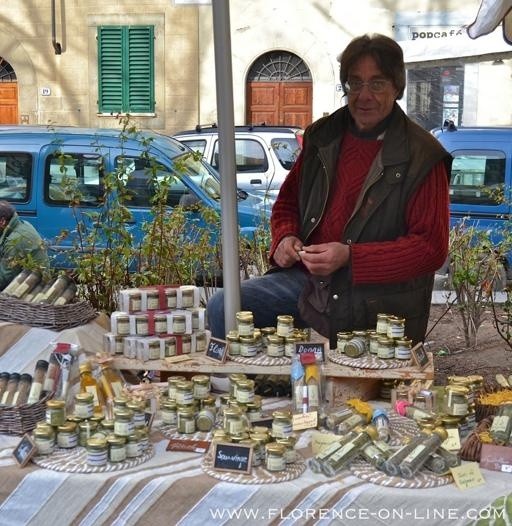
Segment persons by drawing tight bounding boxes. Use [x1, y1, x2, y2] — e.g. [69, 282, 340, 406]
[207, 33, 454, 348]
[0, 200, 50, 291]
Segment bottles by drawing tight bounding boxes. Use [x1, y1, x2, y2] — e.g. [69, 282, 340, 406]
[0, 269, 512, 478]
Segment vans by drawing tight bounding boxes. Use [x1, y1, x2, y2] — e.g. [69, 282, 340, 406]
[0, 126, 273, 273]
[420, 120, 511, 291]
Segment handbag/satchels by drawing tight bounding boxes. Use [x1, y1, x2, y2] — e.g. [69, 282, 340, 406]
[297, 273, 331, 339]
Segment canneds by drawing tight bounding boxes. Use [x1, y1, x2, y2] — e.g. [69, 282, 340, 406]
[0, 267, 512, 478]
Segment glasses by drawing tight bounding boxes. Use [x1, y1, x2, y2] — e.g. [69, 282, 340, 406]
[342, 77, 393, 96]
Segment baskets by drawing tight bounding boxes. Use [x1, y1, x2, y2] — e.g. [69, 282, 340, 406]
[0, 389, 56, 437]
[0, 294, 100, 332]
[474, 386, 512, 421]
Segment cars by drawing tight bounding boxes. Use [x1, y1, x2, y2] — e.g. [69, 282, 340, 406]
[170, 122, 305, 205]
